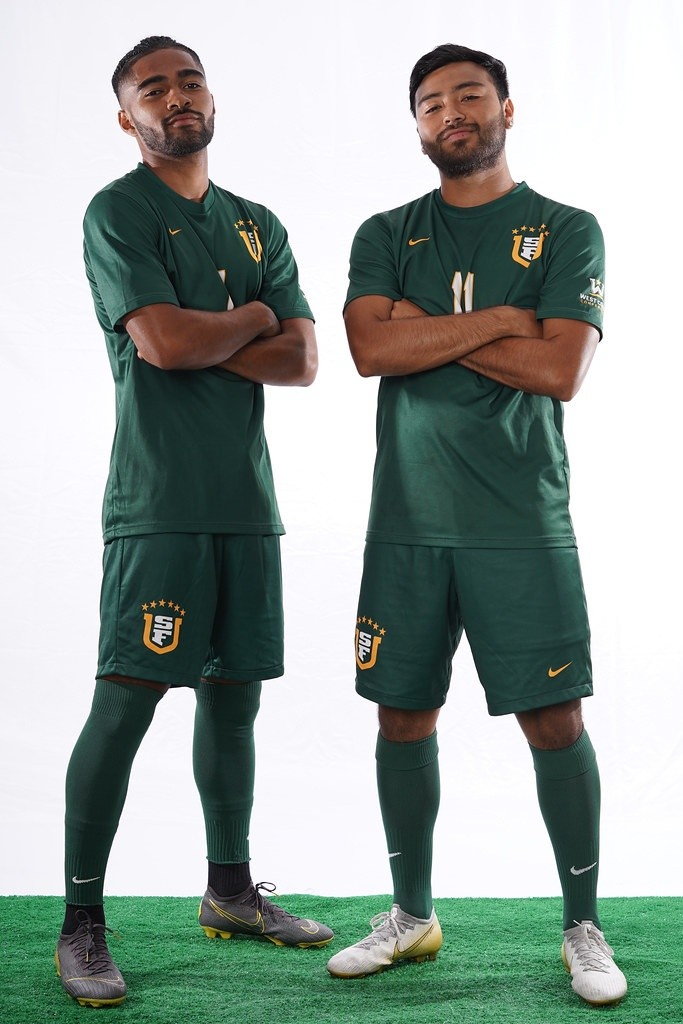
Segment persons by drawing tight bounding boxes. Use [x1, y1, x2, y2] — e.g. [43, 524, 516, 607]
[57, 36, 334, 1008]
[326, 45, 627, 1006]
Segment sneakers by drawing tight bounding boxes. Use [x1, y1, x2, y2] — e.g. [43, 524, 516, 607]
[327, 904, 442, 978]
[198, 884, 336, 948]
[53, 926, 127, 1008]
[560, 919, 627, 1004]
